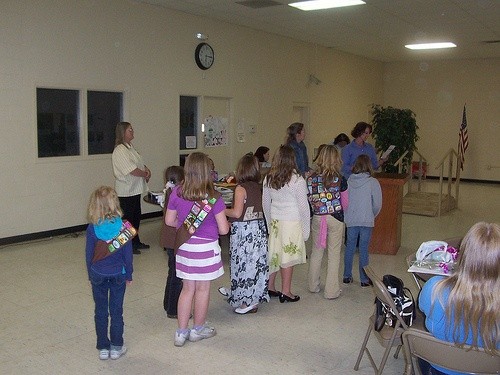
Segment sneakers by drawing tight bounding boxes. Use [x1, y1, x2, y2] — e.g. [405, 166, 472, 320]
[190, 327, 216, 340]
[174, 330, 190, 346]
[110, 346, 126, 359]
[99, 349, 110, 359]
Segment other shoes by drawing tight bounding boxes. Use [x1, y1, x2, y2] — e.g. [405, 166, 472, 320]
[167, 312, 193, 319]
[361, 280, 372, 287]
[133, 249, 140, 254]
[342, 278, 353, 284]
[138, 243, 149, 249]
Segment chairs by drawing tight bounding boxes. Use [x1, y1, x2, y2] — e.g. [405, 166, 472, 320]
[402, 328, 500, 375]
[353, 266, 430, 375]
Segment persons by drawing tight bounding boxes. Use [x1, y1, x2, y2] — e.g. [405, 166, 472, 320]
[159, 166, 193, 319]
[344, 154, 382, 288]
[254, 146, 272, 184]
[286, 122, 313, 179]
[307, 144, 348, 299]
[333, 133, 350, 147]
[86, 186, 133, 360]
[263, 144, 310, 303]
[341, 122, 389, 180]
[218, 154, 270, 314]
[417, 222, 500, 375]
[112, 122, 151, 255]
[165, 152, 232, 347]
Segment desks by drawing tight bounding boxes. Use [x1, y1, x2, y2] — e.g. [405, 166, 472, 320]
[368, 172, 410, 256]
[406, 235, 463, 283]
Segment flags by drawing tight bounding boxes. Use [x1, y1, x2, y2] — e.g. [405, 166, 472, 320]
[458, 105, 469, 171]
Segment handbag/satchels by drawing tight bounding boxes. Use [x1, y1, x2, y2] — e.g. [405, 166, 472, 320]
[374, 275, 415, 331]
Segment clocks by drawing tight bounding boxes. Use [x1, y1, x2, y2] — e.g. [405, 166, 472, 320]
[195, 43, 214, 70]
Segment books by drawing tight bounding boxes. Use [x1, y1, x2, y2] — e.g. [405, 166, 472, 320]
[381, 145, 395, 159]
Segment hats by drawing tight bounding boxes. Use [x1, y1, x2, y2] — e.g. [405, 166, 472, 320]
[408, 240, 457, 276]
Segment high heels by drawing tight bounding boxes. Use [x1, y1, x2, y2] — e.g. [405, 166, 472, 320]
[234, 303, 257, 314]
[218, 287, 228, 296]
[279, 292, 300, 303]
[268, 290, 280, 298]
